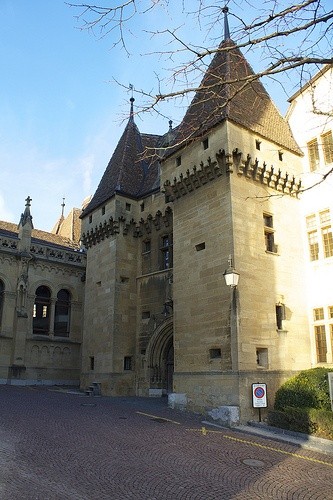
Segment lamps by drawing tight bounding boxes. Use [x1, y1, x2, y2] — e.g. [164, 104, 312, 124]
[223, 252, 240, 288]
[169, 120, 174, 127]
